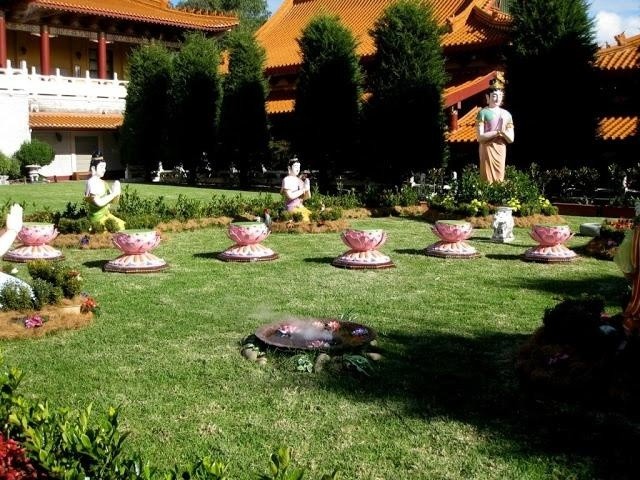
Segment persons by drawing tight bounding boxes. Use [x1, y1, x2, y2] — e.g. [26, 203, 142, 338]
[280, 153, 314, 215]
[0, 202, 40, 301]
[476, 80, 517, 180]
[85, 149, 125, 231]
